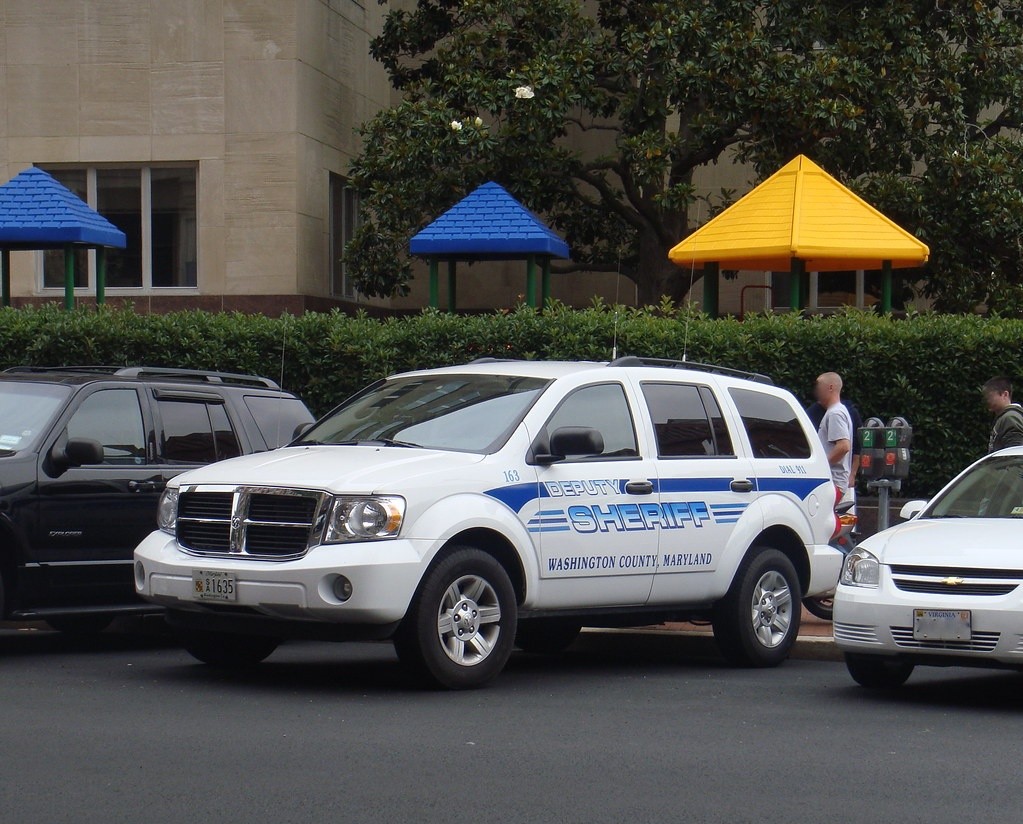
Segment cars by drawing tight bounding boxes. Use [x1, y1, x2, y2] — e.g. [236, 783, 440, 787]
[832, 446, 1023, 689]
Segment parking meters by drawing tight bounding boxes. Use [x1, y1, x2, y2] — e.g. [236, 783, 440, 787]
[857, 416, 912, 532]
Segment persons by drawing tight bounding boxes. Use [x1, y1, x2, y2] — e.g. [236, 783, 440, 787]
[978, 375, 1023, 517]
[806, 401, 864, 532]
[815, 372, 852, 499]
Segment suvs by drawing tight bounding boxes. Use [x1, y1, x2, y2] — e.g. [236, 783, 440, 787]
[132, 353, 845, 691]
[0, 363, 317, 649]
